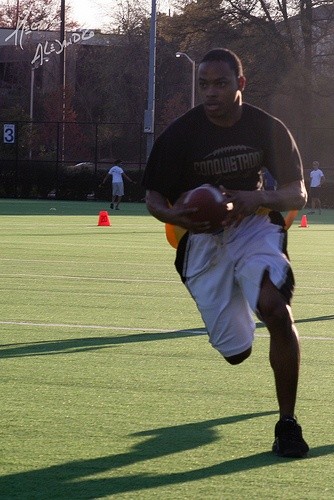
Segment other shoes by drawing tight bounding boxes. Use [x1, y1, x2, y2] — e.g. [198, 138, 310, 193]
[111, 204, 114, 209]
[116, 205, 119, 210]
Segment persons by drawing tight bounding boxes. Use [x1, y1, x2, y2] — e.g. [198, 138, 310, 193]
[262, 167, 277, 191]
[101, 160, 132, 210]
[308, 162, 326, 215]
[145, 49, 311, 456]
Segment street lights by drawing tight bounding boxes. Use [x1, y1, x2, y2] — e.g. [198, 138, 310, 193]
[175, 51, 195, 111]
[29, 56, 50, 159]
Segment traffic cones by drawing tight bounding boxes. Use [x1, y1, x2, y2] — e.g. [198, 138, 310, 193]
[98, 210, 112, 227]
[298, 214, 309, 228]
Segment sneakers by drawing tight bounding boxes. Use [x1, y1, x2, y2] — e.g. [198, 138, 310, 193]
[273, 421, 310, 458]
